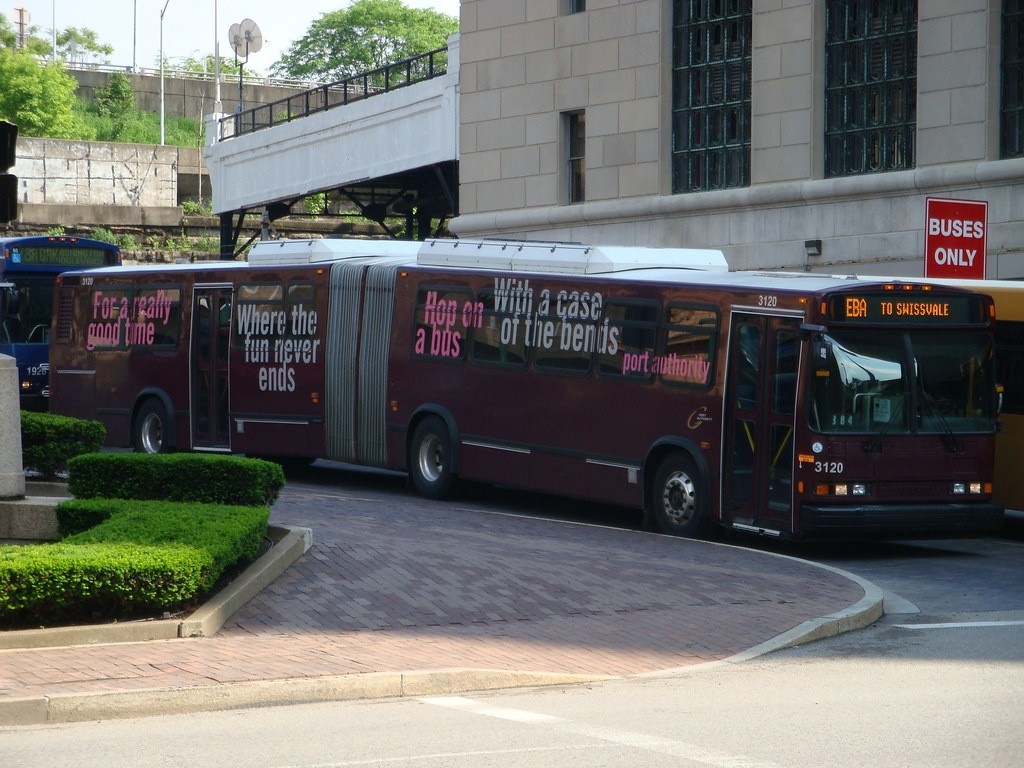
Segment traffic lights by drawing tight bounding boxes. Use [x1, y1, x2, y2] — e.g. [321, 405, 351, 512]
[0, 120, 20, 224]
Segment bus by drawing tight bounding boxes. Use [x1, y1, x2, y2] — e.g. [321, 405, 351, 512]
[833, 271, 1024, 533]
[0, 236, 123, 410]
[49, 239, 1010, 543]
